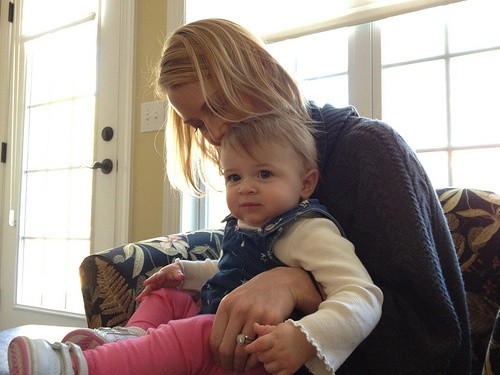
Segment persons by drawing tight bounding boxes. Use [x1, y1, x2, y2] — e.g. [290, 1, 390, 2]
[147, 18, 474, 375]
[7, 111, 384, 375]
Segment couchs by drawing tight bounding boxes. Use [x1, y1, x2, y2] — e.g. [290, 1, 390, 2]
[80, 188, 499, 375]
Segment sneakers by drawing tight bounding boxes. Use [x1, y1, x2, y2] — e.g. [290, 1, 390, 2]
[7, 326, 149, 375]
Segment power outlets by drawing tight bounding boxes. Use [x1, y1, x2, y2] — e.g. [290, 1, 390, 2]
[140, 100, 165, 133]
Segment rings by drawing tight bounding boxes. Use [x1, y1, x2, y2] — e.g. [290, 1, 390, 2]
[236, 334, 256, 345]
